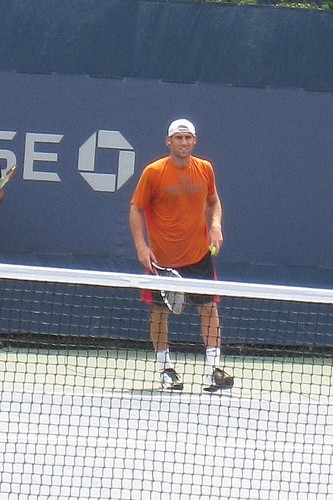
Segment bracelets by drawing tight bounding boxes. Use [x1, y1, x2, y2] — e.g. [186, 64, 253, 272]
[210, 223, 221, 231]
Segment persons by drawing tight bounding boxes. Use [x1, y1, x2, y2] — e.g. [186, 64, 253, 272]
[127, 118, 235, 391]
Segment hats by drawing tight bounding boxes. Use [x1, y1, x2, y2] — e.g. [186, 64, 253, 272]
[167, 119, 196, 136]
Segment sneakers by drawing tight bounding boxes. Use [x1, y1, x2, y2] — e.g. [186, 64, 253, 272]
[203, 368, 233, 392]
[159, 368, 182, 390]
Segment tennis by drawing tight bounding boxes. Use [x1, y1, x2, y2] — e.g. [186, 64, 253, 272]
[209, 246, 217, 257]
